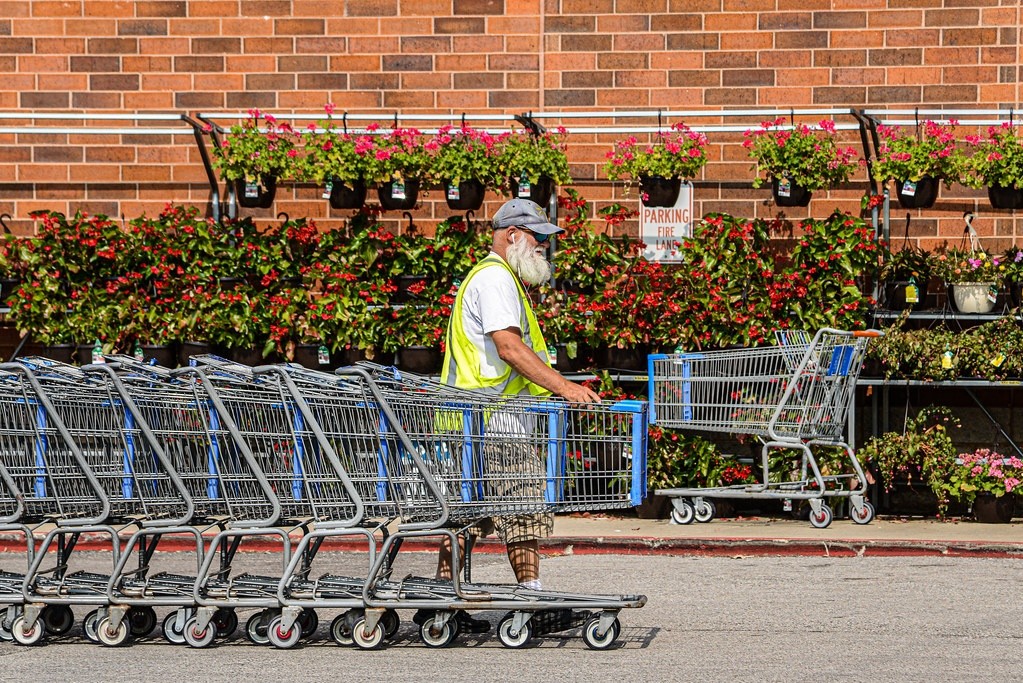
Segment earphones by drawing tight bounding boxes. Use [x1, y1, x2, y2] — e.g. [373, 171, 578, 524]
[511, 233, 515, 243]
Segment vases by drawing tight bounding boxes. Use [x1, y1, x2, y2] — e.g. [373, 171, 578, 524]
[0, 165, 1023, 525]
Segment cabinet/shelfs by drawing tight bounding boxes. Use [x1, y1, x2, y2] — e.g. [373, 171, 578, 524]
[365, 312, 1021, 518]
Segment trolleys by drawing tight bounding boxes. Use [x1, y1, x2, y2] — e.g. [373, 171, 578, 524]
[0, 349, 649, 649]
[647, 324, 889, 528]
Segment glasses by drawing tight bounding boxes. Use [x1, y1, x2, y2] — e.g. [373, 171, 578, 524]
[533, 231, 550, 242]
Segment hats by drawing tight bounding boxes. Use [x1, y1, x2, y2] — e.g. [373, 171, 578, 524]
[492, 197, 565, 235]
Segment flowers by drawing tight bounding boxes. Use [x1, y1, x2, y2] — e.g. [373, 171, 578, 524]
[0, 99, 1023, 524]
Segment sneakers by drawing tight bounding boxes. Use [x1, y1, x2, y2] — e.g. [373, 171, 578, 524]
[413, 605, 490, 635]
[531, 608, 591, 636]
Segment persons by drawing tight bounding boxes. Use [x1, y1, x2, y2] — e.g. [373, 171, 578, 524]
[412, 197, 603, 639]
